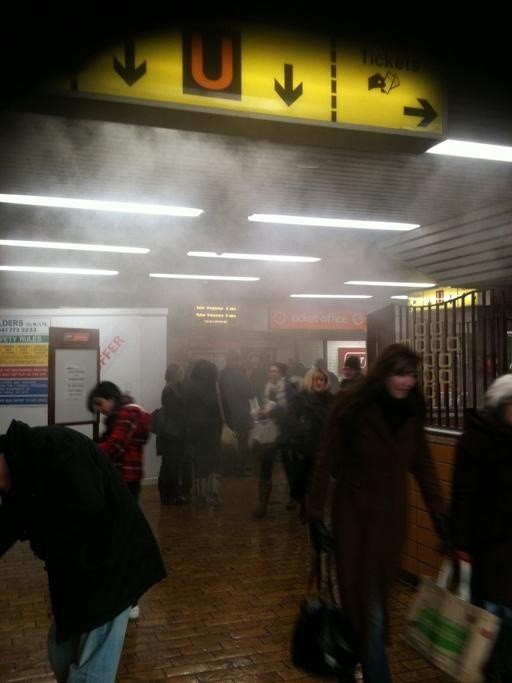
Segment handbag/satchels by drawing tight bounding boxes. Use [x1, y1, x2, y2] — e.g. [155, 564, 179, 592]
[150, 408, 184, 444]
[220, 424, 240, 458]
[247, 419, 282, 452]
[292, 596, 359, 683]
[397, 573, 504, 683]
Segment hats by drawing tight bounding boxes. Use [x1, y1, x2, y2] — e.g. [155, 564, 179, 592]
[482, 374, 512, 409]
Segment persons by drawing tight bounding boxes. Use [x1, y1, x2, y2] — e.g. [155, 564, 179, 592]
[303, 339, 454, 682]
[449, 370, 512, 683]
[155, 345, 364, 528]
[0, 415, 166, 683]
[84, 379, 150, 624]
